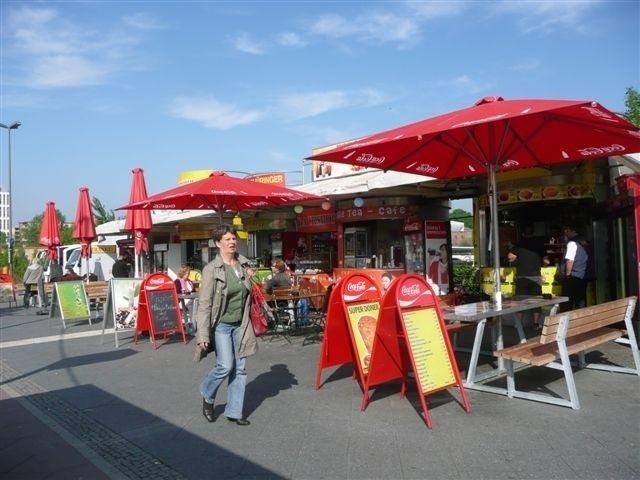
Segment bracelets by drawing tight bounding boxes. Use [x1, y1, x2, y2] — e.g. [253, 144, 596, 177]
[565, 274, 572, 278]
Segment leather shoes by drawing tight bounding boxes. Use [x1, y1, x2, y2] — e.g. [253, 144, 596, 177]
[203, 397, 215, 422]
[227, 416, 250, 425]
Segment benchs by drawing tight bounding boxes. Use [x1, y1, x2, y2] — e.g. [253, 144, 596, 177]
[25, 285, 640, 410]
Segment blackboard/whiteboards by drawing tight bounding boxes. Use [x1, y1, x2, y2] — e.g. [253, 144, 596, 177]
[144, 285, 183, 334]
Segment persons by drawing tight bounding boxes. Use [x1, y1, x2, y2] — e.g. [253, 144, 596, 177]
[62, 267, 78, 277]
[111, 251, 132, 278]
[559, 222, 594, 314]
[171, 266, 199, 315]
[46, 258, 63, 283]
[21, 257, 45, 310]
[242, 247, 311, 332]
[378, 273, 394, 299]
[427, 242, 450, 286]
[507, 220, 551, 330]
[191, 222, 259, 428]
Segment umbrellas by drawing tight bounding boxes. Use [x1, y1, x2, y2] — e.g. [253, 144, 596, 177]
[70, 186, 98, 309]
[304, 92, 640, 369]
[123, 166, 153, 277]
[38, 201, 64, 261]
[112, 169, 332, 227]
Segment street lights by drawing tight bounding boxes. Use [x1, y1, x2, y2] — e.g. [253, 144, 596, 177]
[0, 121, 22, 280]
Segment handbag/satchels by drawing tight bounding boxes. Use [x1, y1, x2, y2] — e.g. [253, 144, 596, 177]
[250, 282, 277, 337]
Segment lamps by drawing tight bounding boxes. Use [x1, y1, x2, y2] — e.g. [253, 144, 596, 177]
[321, 198, 331, 211]
[294, 205, 304, 214]
[353, 196, 365, 209]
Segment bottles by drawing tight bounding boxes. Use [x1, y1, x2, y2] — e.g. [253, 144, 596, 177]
[427, 248, 440, 257]
